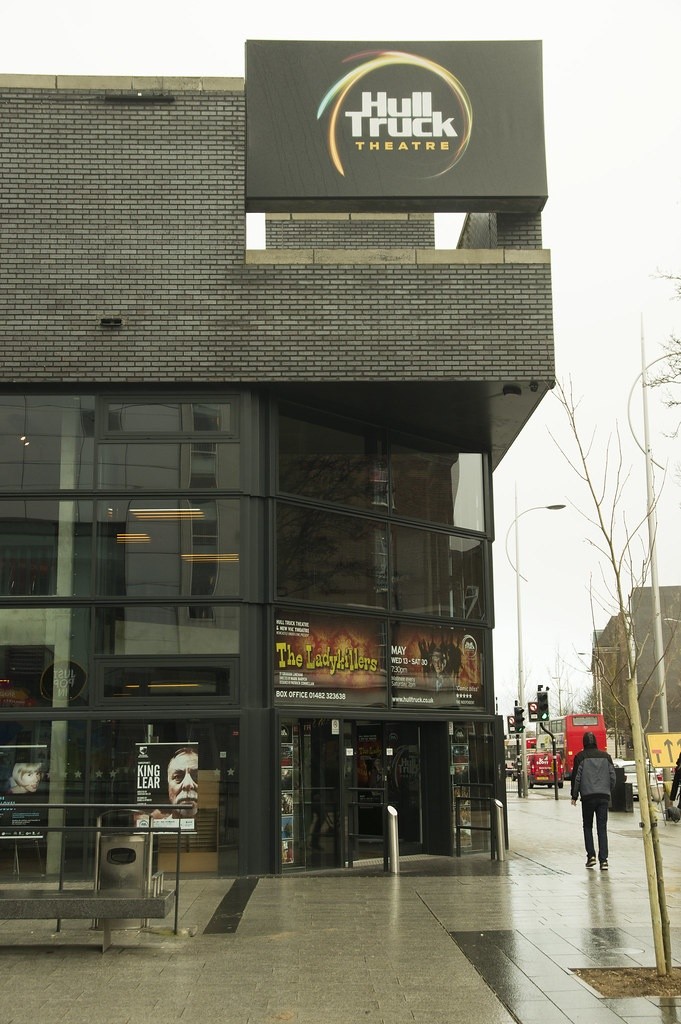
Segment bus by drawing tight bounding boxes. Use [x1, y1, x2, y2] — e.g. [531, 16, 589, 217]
[536, 714, 607, 781]
[505, 734, 537, 775]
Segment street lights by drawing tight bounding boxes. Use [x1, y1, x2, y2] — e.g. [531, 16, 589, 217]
[504, 480, 566, 800]
[578, 651, 604, 715]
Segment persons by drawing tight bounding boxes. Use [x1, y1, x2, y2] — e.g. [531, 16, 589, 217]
[669, 752, 681, 810]
[7, 763, 46, 799]
[432, 650, 453, 691]
[570, 732, 616, 870]
[168, 747, 199, 822]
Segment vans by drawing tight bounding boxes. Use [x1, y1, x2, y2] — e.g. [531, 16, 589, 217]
[526, 751, 563, 788]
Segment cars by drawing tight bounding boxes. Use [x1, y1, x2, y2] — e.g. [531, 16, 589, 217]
[614, 758, 675, 800]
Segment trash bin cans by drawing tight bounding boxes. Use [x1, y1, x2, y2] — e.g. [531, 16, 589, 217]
[613, 767, 634, 813]
[100, 833, 149, 931]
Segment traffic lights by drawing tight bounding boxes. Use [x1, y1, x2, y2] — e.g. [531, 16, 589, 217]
[537, 692, 550, 721]
[514, 706, 525, 733]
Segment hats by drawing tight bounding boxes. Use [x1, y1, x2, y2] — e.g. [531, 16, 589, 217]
[583, 732, 596, 745]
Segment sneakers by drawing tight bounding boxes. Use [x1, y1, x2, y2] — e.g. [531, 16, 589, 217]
[600, 859, 609, 869]
[586, 853, 596, 867]
